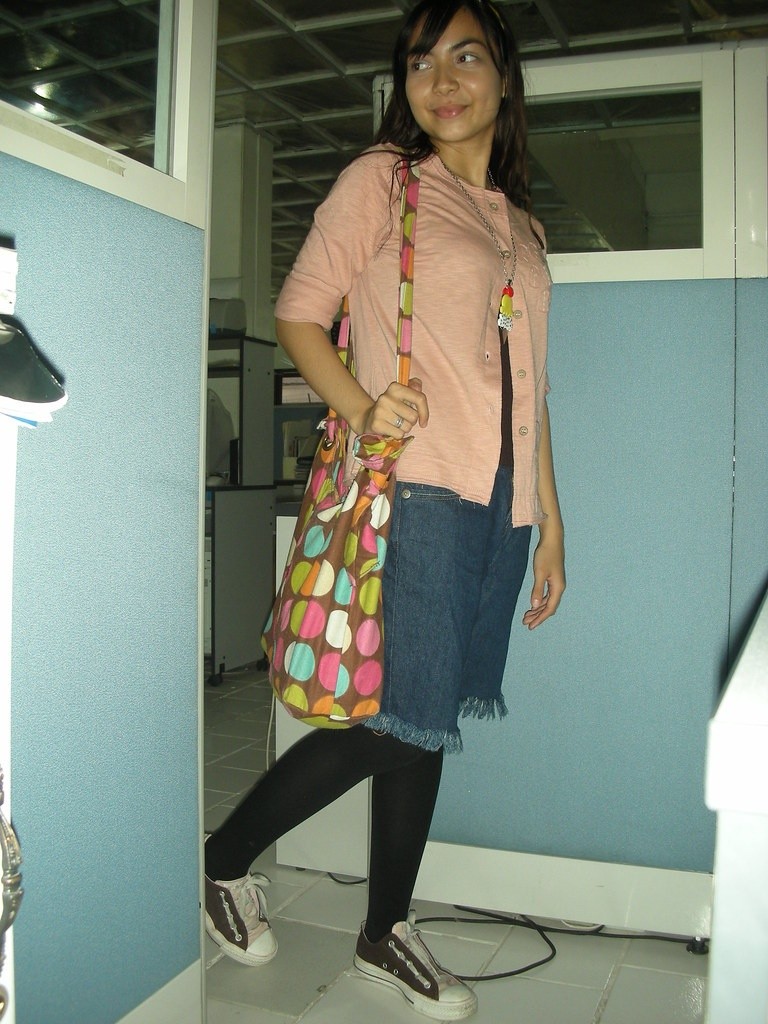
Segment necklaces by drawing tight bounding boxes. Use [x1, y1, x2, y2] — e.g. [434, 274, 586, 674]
[440, 159, 517, 331]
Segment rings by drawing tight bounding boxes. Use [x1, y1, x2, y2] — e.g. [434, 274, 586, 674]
[396, 418, 404, 429]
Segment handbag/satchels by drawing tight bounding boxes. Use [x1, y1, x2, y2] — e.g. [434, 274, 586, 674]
[260, 408, 414, 730]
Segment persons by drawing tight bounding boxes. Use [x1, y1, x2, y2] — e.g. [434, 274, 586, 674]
[205, 1, 565, 1022]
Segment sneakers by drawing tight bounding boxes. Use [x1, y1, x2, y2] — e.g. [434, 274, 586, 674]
[352, 909, 478, 1021]
[202, 833, 279, 967]
